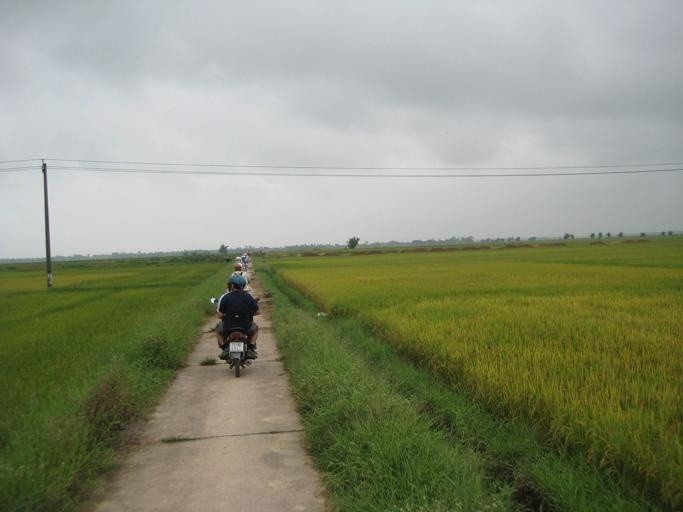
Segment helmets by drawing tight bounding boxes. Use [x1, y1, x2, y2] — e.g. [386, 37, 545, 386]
[228, 253, 247, 285]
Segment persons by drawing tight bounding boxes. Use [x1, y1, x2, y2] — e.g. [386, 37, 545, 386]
[215, 252, 263, 359]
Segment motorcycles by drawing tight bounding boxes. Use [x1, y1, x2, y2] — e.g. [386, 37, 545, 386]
[211, 297, 260, 377]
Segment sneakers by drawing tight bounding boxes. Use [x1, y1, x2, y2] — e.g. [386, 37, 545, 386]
[219, 349, 228, 358]
[246, 349, 257, 357]
